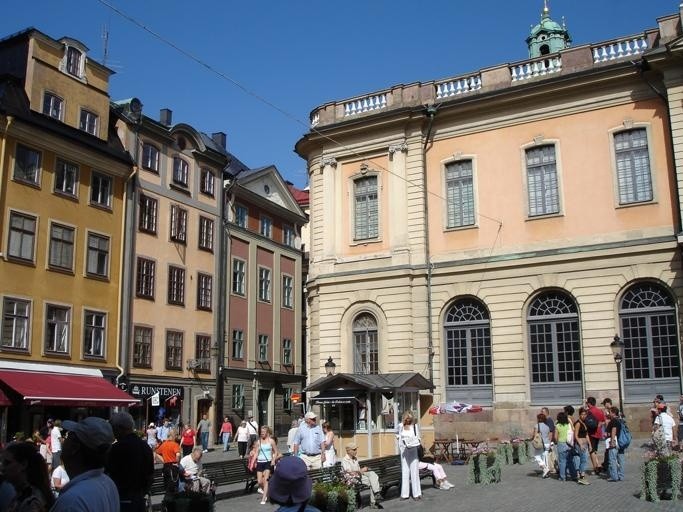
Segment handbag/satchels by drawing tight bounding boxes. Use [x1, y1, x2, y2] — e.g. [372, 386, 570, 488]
[248, 455, 257, 470]
[418, 445, 423, 460]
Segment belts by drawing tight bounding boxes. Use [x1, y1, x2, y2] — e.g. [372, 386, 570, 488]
[303, 453, 321, 456]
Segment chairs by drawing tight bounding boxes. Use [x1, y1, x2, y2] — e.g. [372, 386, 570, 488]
[431, 437, 483, 464]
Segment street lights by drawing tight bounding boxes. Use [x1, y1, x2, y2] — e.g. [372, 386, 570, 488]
[324, 356, 336, 429]
[609, 333, 625, 418]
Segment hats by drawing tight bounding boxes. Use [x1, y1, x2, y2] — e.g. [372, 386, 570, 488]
[346, 443, 359, 450]
[268, 456, 312, 503]
[62, 417, 114, 453]
[305, 412, 318, 419]
[148, 422, 156, 428]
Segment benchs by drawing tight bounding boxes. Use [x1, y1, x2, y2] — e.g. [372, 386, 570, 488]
[143, 452, 294, 512]
[307, 453, 437, 508]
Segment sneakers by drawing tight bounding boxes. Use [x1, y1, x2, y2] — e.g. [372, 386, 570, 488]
[444, 480, 455, 488]
[440, 482, 450, 490]
[375, 492, 384, 500]
[257, 488, 267, 505]
[370, 503, 381, 509]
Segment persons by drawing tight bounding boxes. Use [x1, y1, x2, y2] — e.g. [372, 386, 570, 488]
[533, 397, 625, 485]
[341, 443, 384, 509]
[220, 417, 232, 453]
[287, 412, 336, 487]
[394, 410, 455, 501]
[233, 417, 282, 505]
[0, 409, 216, 512]
[651, 395, 682, 455]
